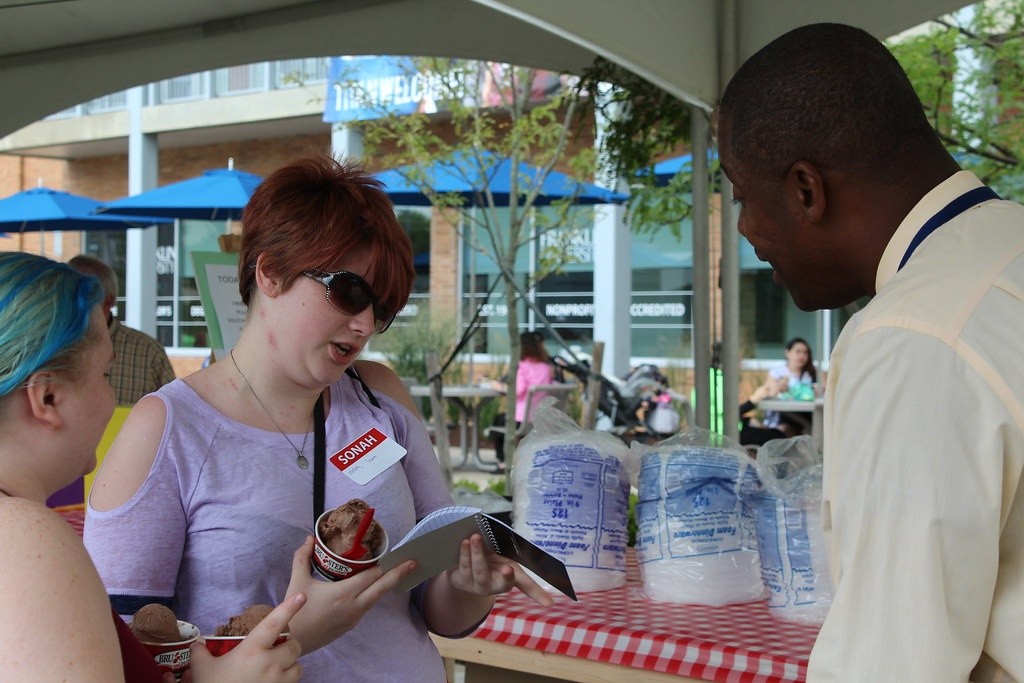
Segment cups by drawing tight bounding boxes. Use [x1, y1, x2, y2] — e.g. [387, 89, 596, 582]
[310, 508, 389, 582]
[203, 632, 290, 657]
[128, 621, 200, 683]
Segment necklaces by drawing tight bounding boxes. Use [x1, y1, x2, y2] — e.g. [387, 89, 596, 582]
[230, 348, 312, 470]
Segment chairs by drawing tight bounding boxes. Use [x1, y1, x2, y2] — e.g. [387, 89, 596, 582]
[665, 388, 761, 458]
[484, 384, 579, 439]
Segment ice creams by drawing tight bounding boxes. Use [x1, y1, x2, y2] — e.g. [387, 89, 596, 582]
[312, 497, 389, 583]
[120, 604, 290, 683]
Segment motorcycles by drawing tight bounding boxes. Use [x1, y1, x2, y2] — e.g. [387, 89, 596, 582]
[558, 354, 669, 448]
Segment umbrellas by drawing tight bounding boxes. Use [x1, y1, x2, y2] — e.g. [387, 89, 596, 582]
[0, 157, 263, 255]
[362, 137, 636, 387]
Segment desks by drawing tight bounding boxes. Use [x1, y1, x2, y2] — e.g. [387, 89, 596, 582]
[408, 387, 505, 471]
[429, 546, 824, 683]
[760, 400, 814, 435]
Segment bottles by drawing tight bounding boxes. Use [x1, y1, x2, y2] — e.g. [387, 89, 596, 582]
[800, 371, 815, 401]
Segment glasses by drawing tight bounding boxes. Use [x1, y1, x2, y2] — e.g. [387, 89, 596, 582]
[303, 269, 403, 335]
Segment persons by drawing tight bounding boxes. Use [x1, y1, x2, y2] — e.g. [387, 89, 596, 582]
[719, 23, 1024, 683]
[82, 146, 555, 683]
[68, 254, 176, 407]
[490, 328, 560, 475]
[0, 255, 313, 683]
[690, 336, 830, 448]
